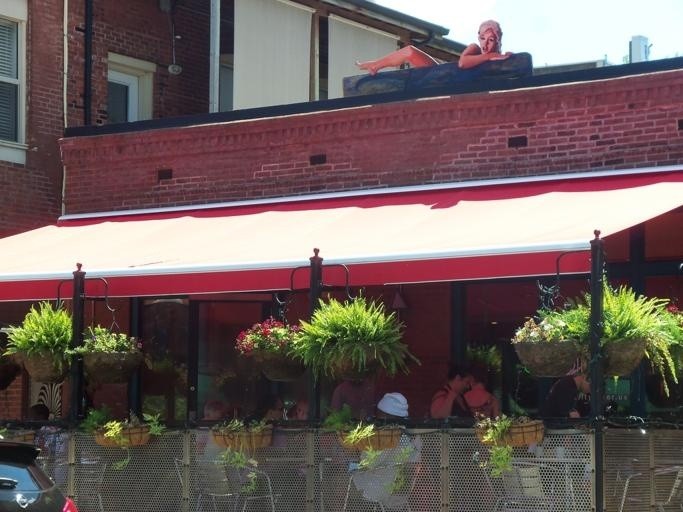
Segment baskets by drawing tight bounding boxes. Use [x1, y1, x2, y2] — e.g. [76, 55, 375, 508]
[213, 430, 273, 449]
[475, 421, 545, 445]
[254, 349, 307, 383]
[94, 424, 154, 447]
[22, 347, 69, 384]
[329, 355, 384, 381]
[336, 432, 401, 451]
[584, 341, 647, 380]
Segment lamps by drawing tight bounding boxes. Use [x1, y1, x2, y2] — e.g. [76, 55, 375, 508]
[158, 1, 182, 75]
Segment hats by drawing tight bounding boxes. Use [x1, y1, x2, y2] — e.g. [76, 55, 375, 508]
[377, 392, 409, 417]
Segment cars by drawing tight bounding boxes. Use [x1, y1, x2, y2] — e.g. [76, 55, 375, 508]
[0, 436, 79, 512]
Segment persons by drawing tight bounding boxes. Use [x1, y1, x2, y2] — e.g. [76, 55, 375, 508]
[429, 370, 500, 418]
[201, 399, 311, 489]
[354, 20, 513, 77]
[542, 375, 596, 419]
[29, 404, 49, 420]
[348, 392, 421, 503]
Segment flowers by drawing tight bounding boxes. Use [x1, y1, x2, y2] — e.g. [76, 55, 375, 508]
[510, 315, 578, 345]
[64, 324, 143, 357]
[232, 316, 309, 358]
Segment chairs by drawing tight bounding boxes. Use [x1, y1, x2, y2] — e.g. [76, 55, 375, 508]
[0, 421, 683, 510]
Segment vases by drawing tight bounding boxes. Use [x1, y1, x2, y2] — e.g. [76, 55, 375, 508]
[245, 351, 285, 382]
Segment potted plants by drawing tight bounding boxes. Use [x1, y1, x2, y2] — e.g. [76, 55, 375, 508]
[1, 298, 72, 382]
[472, 412, 545, 480]
[78, 404, 168, 470]
[535, 276, 683, 398]
[319, 403, 414, 494]
[285, 290, 421, 384]
[210, 418, 273, 496]
